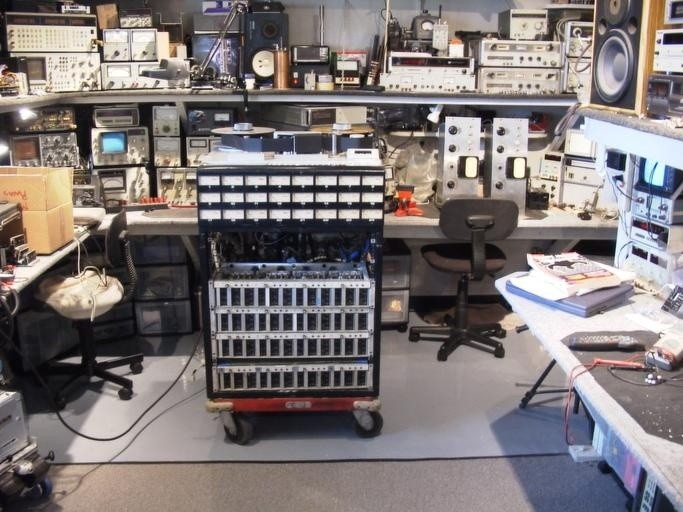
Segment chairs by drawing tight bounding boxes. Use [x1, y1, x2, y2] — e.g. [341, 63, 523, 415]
[34, 212, 144, 412]
[410, 199, 519, 362]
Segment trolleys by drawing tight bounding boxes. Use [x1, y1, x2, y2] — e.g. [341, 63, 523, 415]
[196, 146, 382, 446]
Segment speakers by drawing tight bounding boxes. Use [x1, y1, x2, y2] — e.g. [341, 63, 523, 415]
[244, 11, 289, 85]
[589, 0, 666, 118]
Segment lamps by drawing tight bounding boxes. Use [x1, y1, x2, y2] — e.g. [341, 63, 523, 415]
[137, 2, 247, 110]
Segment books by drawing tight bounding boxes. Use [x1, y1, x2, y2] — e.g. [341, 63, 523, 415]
[528, 251, 619, 296]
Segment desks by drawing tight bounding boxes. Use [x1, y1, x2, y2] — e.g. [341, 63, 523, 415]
[2, 89, 616, 357]
[496, 265, 681, 512]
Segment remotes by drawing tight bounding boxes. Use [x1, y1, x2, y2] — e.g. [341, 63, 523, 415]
[568, 335, 645, 350]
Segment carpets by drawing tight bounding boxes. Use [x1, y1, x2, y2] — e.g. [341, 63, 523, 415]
[24, 327, 593, 464]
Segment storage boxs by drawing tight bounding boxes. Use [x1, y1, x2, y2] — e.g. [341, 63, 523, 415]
[135, 264, 190, 301]
[0, 165, 75, 255]
[135, 303, 192, 336]
[380, 289, 409, 331]
[134, 232, 188, 265]
[380, 237, 409, 289]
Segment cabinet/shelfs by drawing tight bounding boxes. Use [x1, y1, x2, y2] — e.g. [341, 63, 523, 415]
[577, 1, 682, 302]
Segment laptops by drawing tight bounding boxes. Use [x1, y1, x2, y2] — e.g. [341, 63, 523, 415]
[506, 271, 635, 318]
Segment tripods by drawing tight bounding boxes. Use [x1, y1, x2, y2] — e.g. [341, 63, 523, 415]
[518, 358, 595, 442]
